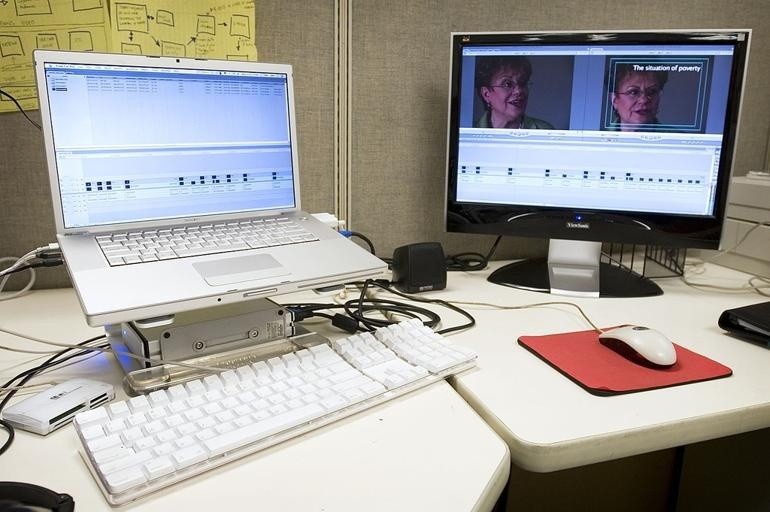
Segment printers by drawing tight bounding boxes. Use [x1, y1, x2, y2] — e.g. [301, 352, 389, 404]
[700, 170, 770, 279]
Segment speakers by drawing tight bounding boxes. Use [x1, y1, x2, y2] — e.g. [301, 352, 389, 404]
[392, 242, 447, 294]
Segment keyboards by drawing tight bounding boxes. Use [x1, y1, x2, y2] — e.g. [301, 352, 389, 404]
[70, 318, 479, 507]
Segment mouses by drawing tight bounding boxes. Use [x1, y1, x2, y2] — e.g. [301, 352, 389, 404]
[598, 325, 678, 370]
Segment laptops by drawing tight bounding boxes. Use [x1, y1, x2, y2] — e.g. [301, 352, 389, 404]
[33, 49, 389, 327]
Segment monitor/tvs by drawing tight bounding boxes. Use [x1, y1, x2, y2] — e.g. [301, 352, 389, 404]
[445, 29, 754, 299]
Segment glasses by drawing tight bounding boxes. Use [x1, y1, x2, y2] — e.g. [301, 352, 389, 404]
[488, 79, 535, 91]
[613, 87, 661, 98]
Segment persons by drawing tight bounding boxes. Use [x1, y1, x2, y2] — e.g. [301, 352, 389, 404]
[600, 61, 670, 132]
[474, 55, 556, 131]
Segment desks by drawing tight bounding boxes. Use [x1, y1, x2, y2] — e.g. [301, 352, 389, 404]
[0, 287, 512, 512]
[357, 250, 770, 512]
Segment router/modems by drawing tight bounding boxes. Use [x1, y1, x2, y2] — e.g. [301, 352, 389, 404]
[2, 378, 115, 437]
